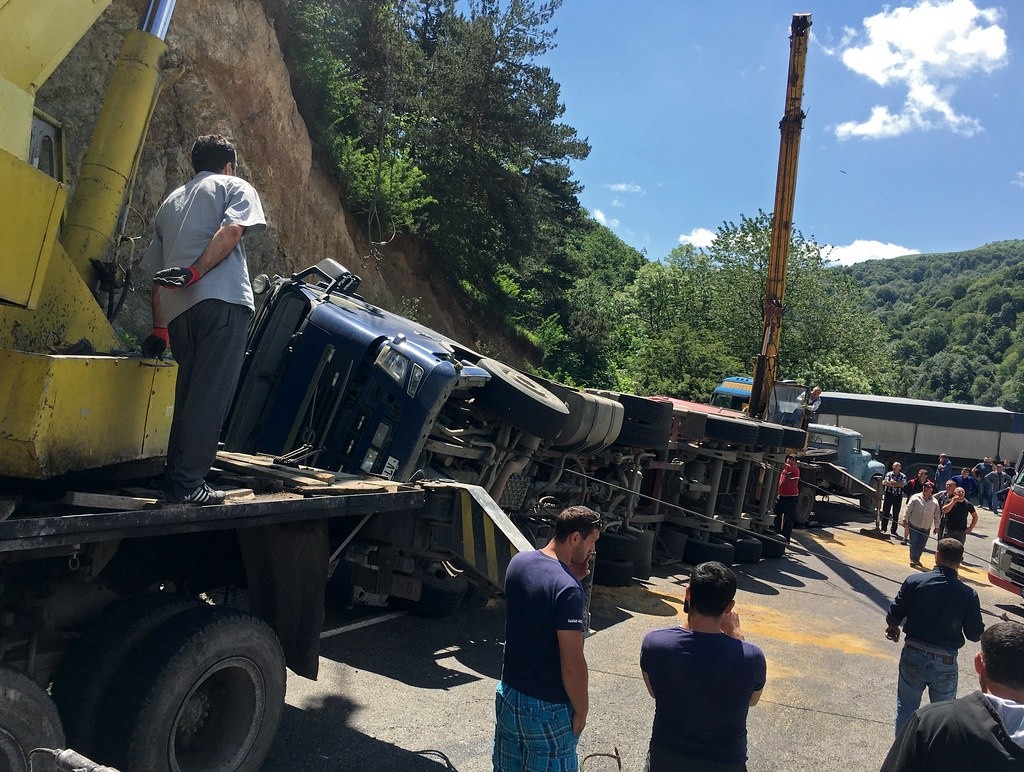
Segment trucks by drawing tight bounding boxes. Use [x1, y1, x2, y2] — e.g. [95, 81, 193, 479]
[794, 416, 887, 523]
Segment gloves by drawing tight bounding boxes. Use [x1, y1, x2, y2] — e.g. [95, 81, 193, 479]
[140, 327, 168, 361]
[800, 402, 808, 409]
[152, 266, 202, 290]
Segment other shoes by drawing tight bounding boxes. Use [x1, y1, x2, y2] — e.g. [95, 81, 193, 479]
[891, 531, 898, 536]
[880, 528, 886, 533]
[978, 504, 981, 508]
[900, 539, 908, 545]
[910, 561, 915, 566]
[989, 508, 993, 511]
[994, 511, 998, 514]
[914, 561, 922, 566]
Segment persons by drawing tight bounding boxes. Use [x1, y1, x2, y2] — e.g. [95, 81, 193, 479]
[971, 456, 1015, 515]
[903, 470, 978, 566]
[492, 506, 605, 772]
[886, 538, 984, 738]
[772, 454, 799, 542]
[934, 454, 953, 492]
[880, 462, 907, 535]
[141, 136, 266, 505]
[640, 561, 766, 772]
[796, 386, 822, 428]
[950, 468, 978, 502]
[879, 622, 1024, 772]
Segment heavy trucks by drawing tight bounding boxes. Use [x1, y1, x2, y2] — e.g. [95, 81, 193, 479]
[0, 0, 885, 772]
[705, 376, 1024, 484]
[988, 450, 1024, 598]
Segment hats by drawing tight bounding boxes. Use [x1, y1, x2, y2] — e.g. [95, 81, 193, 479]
[924, 481, 935, 489]
[790, 453, 798, 462]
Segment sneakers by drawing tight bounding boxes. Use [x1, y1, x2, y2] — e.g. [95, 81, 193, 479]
[167, 482, 227, 506]
[142, 472, 176, 493]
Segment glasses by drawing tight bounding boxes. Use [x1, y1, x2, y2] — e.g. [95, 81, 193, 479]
[589, 514, 603, 527]
